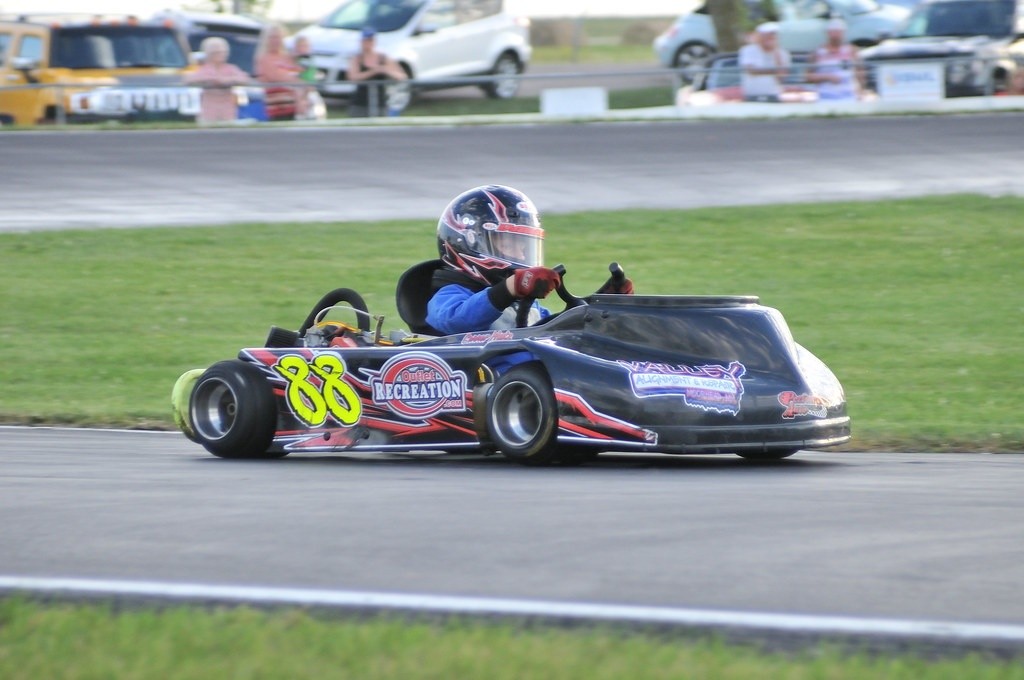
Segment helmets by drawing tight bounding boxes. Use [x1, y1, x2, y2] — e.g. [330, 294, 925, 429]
[435, 184, 544, 286]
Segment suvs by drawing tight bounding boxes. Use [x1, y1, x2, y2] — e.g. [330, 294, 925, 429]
[284, 0, 532, 114]
[0, 13, 264, 129]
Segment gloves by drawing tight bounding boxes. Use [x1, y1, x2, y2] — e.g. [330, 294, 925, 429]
[596, 277, 635, 294]
[515, 266, 561, 300]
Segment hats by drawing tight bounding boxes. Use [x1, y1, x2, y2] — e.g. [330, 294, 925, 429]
[756, 20, 778, 33]
[361, 26, 378, 38]
[822, 17, 845, 30]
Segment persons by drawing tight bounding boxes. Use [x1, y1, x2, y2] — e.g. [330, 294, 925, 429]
[424, 185, 635, 334]
[737, 22, 792, 101]
[184, 36, 256, 121]
[348, 26, 407, 118]
[261, 25, 326, 121]
[293, 37, 312, 70]
[806, 18, 866, 101]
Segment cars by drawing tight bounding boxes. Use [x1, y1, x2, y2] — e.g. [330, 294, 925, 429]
[653, 0, 1024, 106]
